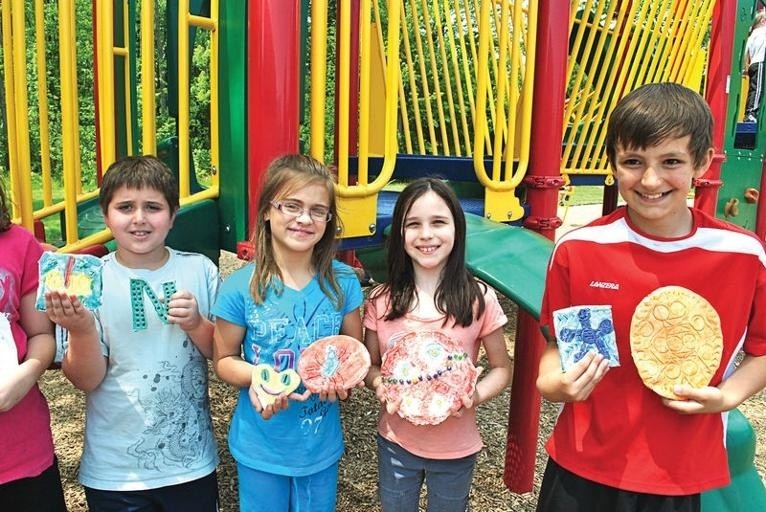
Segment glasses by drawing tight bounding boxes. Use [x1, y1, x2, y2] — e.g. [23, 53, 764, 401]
[268, 199, 333, 224]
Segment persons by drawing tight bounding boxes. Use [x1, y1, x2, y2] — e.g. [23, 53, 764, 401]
[208, 153, 366, 511]
[744, 12, 766, 122]
[44, 155, 224, 512]
[0, 174, 68, 510]
[533, 82, 766, 511]
[358, 176, 512, 511]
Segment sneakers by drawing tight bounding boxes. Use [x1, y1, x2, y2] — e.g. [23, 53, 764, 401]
[743, 114, 756, 122]
